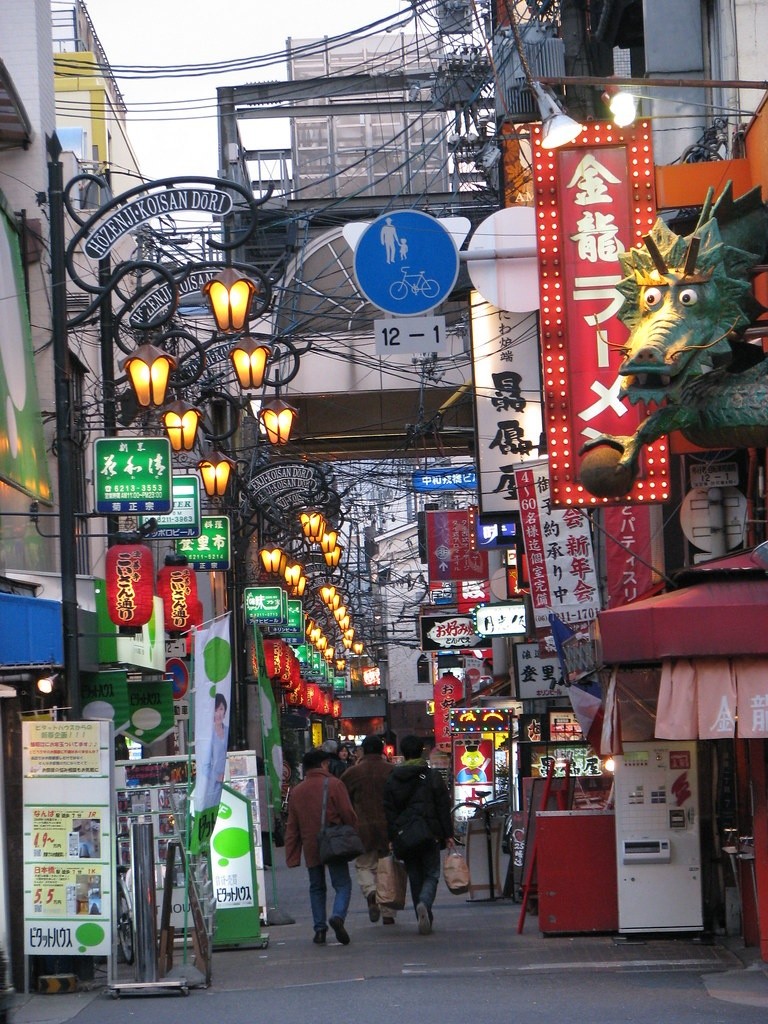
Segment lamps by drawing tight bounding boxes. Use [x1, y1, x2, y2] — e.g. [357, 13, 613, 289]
[601, 85, 640, 126]
[37, 674, 59, 693]
[533, 86, 583, 149]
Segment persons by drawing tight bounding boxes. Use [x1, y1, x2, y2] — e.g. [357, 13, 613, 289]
[204, 693, 229, 808]
[282, 733, 456, 946]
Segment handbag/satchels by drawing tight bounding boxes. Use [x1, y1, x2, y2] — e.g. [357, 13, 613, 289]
[317, 825, 365, 865]
[443, 839, 470, 895]
[376, 850, 408, 910]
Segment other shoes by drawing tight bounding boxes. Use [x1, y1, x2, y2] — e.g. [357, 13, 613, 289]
[367, 891, 380, 922]
[416, 901, 433, 935]
[313, 930, 326, 944]
[328, 917, 351, 946]
[383, 917, 395, 925]
